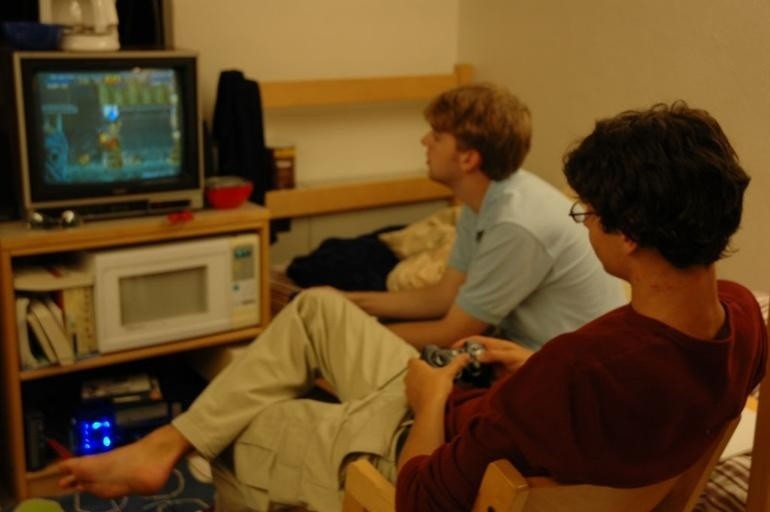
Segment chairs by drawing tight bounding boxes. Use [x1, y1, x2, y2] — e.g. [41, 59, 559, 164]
[337, 410, 741, 512]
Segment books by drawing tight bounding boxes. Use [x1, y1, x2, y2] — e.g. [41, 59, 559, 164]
[14, 266, 96, 369]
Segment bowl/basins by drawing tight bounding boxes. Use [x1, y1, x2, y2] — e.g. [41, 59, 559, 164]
[206, 177, 253, 210]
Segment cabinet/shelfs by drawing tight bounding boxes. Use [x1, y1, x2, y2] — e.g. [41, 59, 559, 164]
[0, 199, 271, 499]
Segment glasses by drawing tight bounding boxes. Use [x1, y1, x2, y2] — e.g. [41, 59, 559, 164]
[568, 201, 601, 226]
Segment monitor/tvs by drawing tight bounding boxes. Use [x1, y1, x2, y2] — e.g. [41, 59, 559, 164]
[13, 51, 204, 223]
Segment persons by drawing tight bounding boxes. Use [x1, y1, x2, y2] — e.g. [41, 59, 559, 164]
[57, 100, 764, 512]
[301, 82, 628, 369]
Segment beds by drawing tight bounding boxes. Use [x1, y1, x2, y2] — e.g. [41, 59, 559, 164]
[203, 62, 770, 510]
